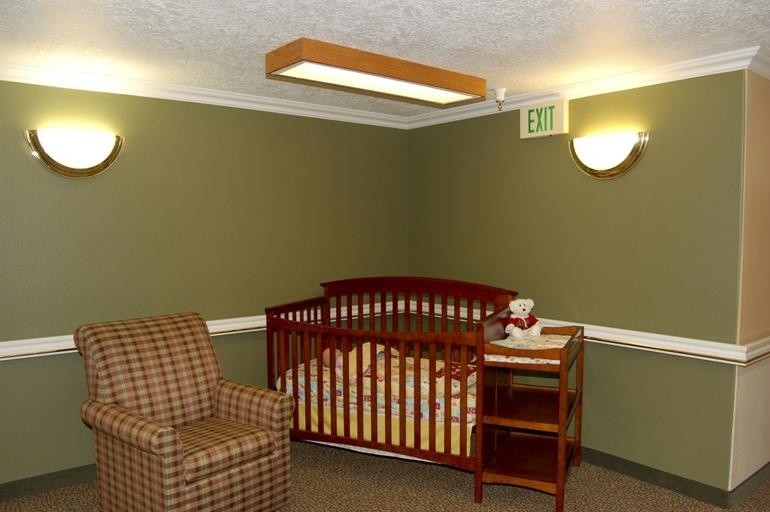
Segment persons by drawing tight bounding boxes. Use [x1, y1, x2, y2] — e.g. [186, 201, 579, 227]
[321, 349, 384, 385]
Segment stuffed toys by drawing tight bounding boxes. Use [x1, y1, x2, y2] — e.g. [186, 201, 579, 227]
[505, 298, 543, 338]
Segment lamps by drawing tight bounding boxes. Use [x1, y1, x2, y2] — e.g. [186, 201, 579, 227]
[27, 126, 126, 178]
[493, 87, 506, 112]
[569, 132, 650, 179]
[265, 37, 487, 110]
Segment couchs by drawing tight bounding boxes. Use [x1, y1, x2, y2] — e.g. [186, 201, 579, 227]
[74, 311, 297, 512]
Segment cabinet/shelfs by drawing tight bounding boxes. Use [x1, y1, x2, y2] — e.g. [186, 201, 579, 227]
[475, 325, 584, 512]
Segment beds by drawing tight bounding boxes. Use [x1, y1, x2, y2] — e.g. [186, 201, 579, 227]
[266, 275, 519, 473]
